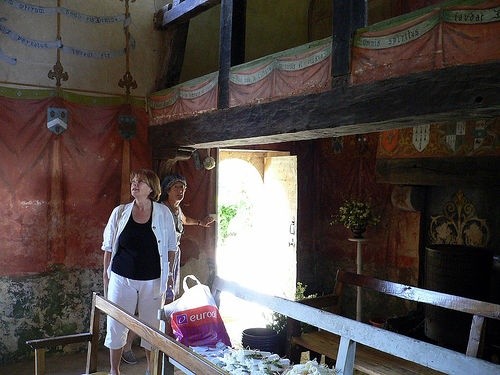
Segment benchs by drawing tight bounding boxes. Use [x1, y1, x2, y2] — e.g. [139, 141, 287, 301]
[24, 269, 500, 375]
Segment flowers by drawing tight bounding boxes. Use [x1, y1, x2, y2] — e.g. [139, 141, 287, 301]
[329, 198, 381, 228]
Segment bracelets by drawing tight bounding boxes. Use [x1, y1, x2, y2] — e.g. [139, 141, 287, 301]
[197, 220, 201, 226]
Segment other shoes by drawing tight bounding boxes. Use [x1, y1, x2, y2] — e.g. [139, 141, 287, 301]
[122, 350, 137, 364]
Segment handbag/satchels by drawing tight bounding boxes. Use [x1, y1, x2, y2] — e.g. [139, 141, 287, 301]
[162, 273, 232, 347]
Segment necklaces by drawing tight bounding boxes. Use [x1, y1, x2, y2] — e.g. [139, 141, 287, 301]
[168, 200, 178, 217]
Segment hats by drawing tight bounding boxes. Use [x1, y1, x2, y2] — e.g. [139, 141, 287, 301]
[162, 176, 187, 195]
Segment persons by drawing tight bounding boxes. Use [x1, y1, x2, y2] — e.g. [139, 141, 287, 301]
[101, 168, 179, 375]
[121, 173, 217, 365]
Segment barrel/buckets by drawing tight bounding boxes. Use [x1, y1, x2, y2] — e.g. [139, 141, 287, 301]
[424, 244, 484, 344]
[242, 328, 280, 356]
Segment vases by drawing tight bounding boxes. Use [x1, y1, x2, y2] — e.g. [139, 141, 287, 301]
[351, 226, 366, 239]
